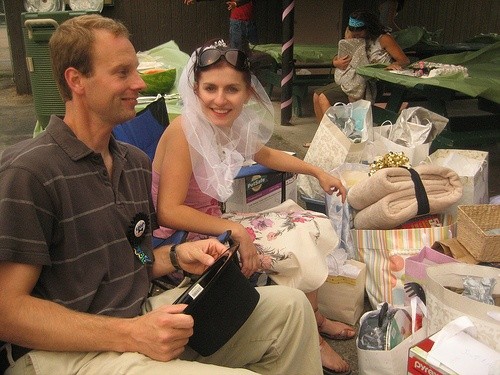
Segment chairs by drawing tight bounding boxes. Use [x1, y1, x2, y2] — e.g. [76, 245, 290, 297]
[112, 96, 295, 299]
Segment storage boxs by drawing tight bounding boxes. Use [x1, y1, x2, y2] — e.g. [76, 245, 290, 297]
[407, 326, 500, 375]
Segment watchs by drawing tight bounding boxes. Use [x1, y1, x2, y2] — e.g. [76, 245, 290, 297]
[169, 244, 182, 270]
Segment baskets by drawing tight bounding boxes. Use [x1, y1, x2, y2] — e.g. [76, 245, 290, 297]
[457, 204, 500, 263]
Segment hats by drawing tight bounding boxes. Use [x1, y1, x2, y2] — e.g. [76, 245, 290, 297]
[172, 242, 260, 357]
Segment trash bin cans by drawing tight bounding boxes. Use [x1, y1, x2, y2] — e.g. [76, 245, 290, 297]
[20, 9, 101, 129]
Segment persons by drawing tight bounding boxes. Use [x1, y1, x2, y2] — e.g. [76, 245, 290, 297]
[184, 0, 258, 50]
[0, 17, 324, 375]
[302, 9, 409, 148]
[151, 34, 358, 375]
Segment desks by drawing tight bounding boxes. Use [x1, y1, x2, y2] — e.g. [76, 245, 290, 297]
[132, 53, 192, 122]
[354, 51, 500, 132]
[250, 44, 490, 119]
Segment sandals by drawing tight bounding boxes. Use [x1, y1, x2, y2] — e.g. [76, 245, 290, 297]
[320, 338, 351, 375]
[318, 315, 355, 339]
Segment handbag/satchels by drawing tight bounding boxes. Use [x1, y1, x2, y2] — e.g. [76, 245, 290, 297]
[318, 254, 367, 326]
[294, 98, 449, 200]
[353, 226, 500, 372]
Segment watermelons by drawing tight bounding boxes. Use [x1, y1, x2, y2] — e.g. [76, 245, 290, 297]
[138, 68, 176, 95]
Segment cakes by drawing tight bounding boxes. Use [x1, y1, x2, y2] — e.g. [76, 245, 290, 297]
[409, 61, 467, 78]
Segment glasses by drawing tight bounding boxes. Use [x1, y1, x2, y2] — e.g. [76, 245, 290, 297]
[197, 49, 251, 72]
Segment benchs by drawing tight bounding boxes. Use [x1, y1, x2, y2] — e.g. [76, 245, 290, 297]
[260, 63, 500, 149]
